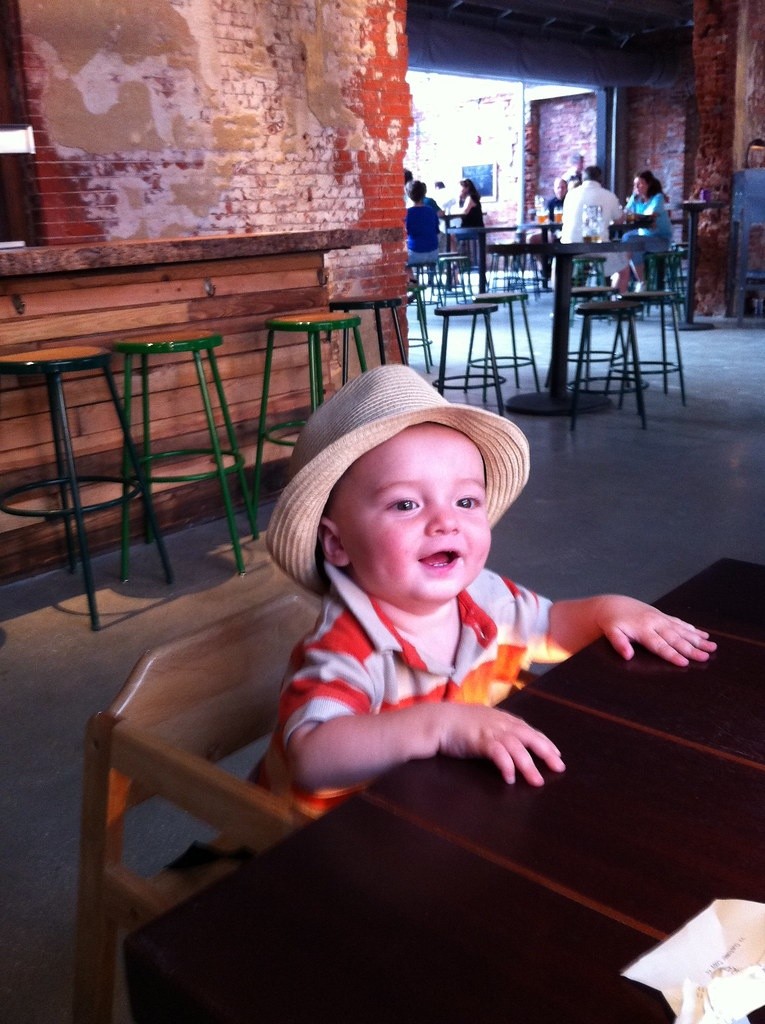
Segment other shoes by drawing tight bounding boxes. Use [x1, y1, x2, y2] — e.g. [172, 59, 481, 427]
[634, 280, 648, 293]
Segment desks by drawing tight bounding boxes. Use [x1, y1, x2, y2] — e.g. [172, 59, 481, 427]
[121, 556, 765, 1024]
[447, 225, 518, 294]
[435, 212, 467, 297]
[0, 226, 404, 294]
[470, 212, 488, 271]
[484, 240, 671, 418]
[517, 224, 563, 293]
[662, 201, 728, 331]
[607, 222, 652, 242]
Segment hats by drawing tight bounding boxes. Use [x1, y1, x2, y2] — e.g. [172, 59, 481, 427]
[265, 364, 530, 596]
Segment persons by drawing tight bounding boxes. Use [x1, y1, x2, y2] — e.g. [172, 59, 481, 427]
[560, 166, 628, 321]
[526, 179, 567, 280]
[405, 181, 441, 285]
[623, 170, 674, 292]
[562, 155, 585, 192]
[404, 167, 483, 286]
[217, 364, 717, 858]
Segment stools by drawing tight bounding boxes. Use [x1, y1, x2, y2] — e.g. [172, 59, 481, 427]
[0, 345, 175, 633]
[249, 314, 369, 521]
[110, 328, 260, 585]
[329, 295, 409, 387]
[407, 241, 689, 430]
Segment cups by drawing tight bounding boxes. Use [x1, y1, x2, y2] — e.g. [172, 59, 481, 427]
[554, 206, 564, 223]
[582, 204, 603, 244]
[536, 206, 549, 224]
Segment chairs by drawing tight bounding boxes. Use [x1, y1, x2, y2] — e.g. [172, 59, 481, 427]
[70, 593, 319, 1024]
